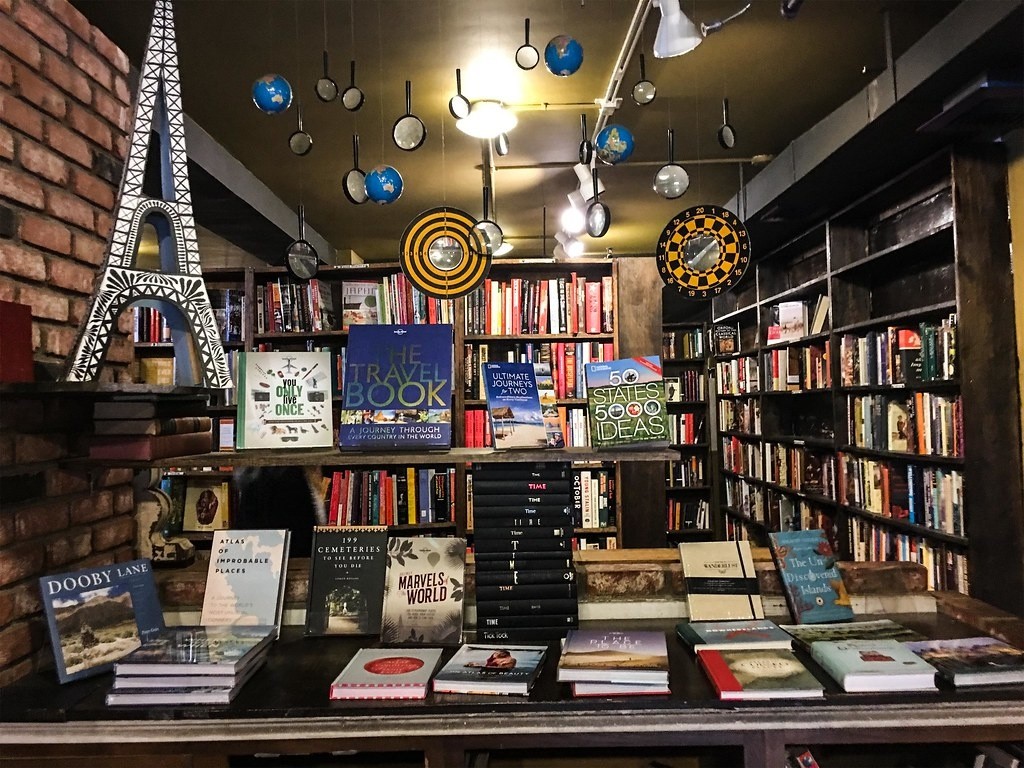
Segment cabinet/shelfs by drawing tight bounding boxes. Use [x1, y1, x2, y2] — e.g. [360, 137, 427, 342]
[0, 138, 1024, 768]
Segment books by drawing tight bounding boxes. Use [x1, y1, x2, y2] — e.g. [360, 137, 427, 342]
[104, 273, 1024, 707]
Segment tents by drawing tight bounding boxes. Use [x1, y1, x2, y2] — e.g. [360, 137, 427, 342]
[490, 407, 515, 439]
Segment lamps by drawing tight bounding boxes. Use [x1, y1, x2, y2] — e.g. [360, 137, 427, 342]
[651, 0, 703, 61]
[491, 241, 514, 257]
[554, 230, 584, 258]
[567, 190, 589, 217]
[573, 162, 607, 203]
[454, 98, 519, 139]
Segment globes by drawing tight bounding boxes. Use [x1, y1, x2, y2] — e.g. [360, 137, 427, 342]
[251, 73, 294, 115]
[363, 165, 405, 205]
[544, 35, 585, 77]
[595, 124, 636, 165]
